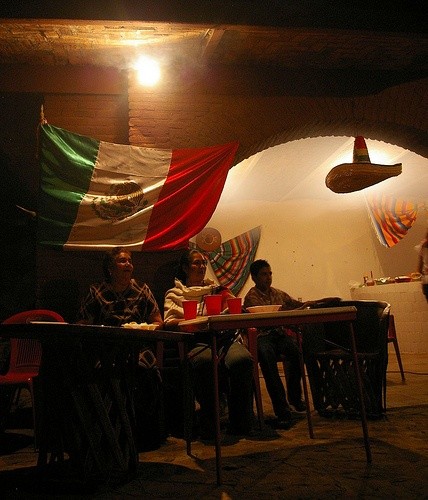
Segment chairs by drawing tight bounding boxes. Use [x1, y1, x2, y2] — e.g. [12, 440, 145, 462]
[0, 309, 65, 436]
[388, 315, 406, 384]
[302, 301, 391, 418]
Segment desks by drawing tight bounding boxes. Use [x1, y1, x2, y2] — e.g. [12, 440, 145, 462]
[178, 306, 373, 485]
[0, 314, 194, 483]
[350, 280, 428, 375]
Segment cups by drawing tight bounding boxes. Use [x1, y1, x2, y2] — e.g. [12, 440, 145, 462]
[182, 300, 198, 320]
[203, 295, 223, 316]
[227, 298, 243, 314]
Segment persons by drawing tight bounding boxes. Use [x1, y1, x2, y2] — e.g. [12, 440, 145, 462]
[243, 260, 341, 429]
[76, 246, 163, 332]
[416, 226, 428, 303]
[163, 249, 265, 441]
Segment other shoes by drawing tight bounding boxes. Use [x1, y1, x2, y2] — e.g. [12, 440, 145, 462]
[288, 397, 306, 411]
[278, 409, 305, 420]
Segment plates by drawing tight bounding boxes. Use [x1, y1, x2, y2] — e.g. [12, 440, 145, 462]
[245, 304, 282, 313]
[396, 277, 412, 282]
[377, 279, 396, 285]
[122, 324, 159, 331]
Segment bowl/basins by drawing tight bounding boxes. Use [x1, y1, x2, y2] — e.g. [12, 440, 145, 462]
[366, 279, 375, 286]
